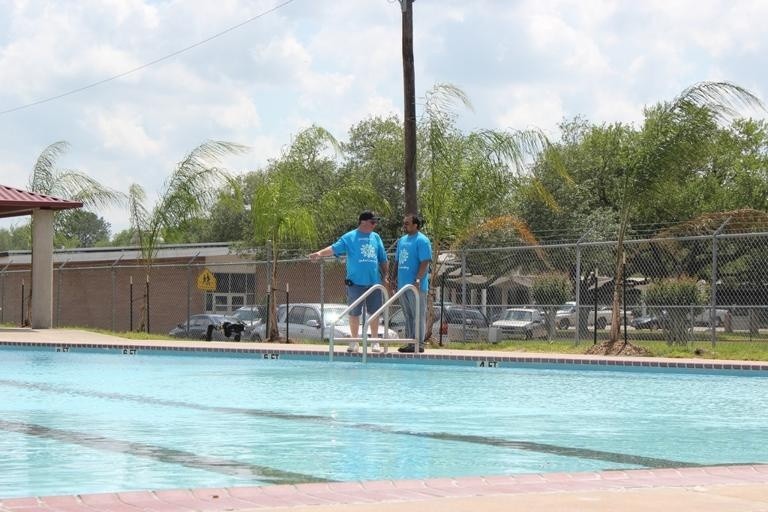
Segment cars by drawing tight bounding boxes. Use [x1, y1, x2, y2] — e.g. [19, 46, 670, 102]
[169, 314, 249, 340]
[629, 310, 691, 331]
[602, 307, 632, 324]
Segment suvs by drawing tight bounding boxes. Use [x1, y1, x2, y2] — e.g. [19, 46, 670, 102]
[378, 302, 490, 345]
[276, 304, 396, 344]
[231, 304, 267, 342]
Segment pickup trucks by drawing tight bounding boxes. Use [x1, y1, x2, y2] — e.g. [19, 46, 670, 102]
[539, 301, 612, 330]
[491, 307, 549, 341]
[686, 305, 729, 326]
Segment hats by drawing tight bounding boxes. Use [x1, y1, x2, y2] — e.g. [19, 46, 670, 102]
[359, 212, 380, 220]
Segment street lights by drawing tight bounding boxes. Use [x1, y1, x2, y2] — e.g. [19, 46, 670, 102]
[622, 251, 628, 346]
[438, 258, 472, 348]
[146, 275, 151, 333]
[587, 272, 599, 344]
[21, 278, 26, 326]
[286, 282, 290, 343]
[266, 284, 270, 339]
[129, 275, 134, 329]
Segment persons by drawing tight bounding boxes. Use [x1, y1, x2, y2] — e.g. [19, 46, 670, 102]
[309, 211, 390, 353]
[390, 214, 432, 353]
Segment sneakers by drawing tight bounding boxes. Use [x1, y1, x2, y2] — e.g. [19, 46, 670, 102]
[347, 341, 424, 352]
